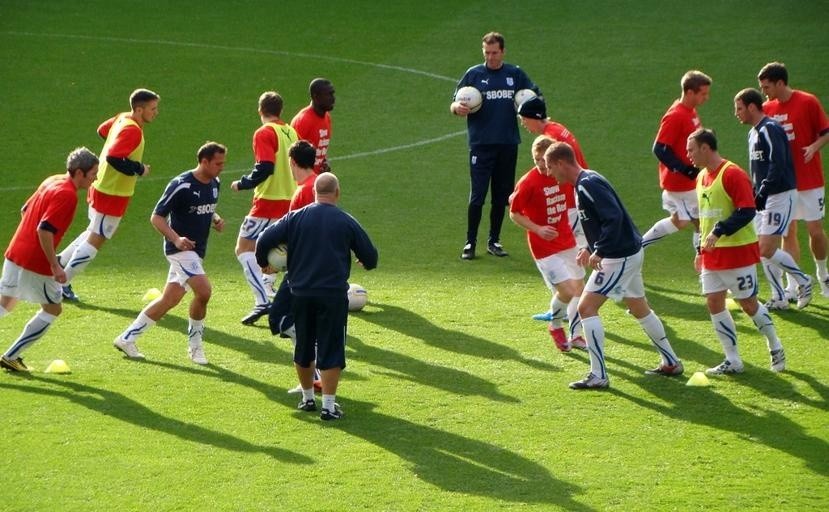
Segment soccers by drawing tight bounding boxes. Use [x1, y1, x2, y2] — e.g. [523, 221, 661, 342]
[266, 242, 289, 272]
[514, 90, 537, 110]
[346, 282, 368, 311]
[455, 86, 483, 114]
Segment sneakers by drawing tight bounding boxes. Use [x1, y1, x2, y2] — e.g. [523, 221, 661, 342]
[0, 354, 30, 372]
[320, 403, 344, 420]
[487, 236, 509, 257]
[114, 336, 146, 360]
[548, 322, 571, 352]
[797, 274, 814, 310]
[297, 398, 317, 412]
[241, 299, 273, 325]
[769, 345, 786, 372]
[645, 359, 684, 376]
[462, 239, 476, 259]
[816, 271, 829, 295]
[532, 311, 569, 321]
[705, 359, 744, 376]
[764, 298, 790, 311]
[61, 284, 78, 300]
[288, 380, 322, 393]
[265, 283, 277, 298]
[784, 288, 798, 303]
[188, 345, 209, 365]
[569, 372, 609, 389]
[568, 335, 588, 351]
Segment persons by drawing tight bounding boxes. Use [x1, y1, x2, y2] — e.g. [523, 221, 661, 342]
[256, 172, 377, 421]
[642, 70, 711, 249]
[758, 63, 829, 296]
[56, 88, 159, 299]
[231, 90, 298, 325]
[734, 88, 814, 310]
[289, 141, 322, 392]
[451, 32, 543, 259]
[544, 141, 683, 391]
[262, 78, 332, 297]
[687, 128, 786, 375]
[112, 142, 224, 366]
[516, 95, 588, 322]
[1, 147, 99, 371]
[510, 135, 588, 352]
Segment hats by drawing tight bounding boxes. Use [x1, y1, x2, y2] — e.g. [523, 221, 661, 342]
[517, 96, 547, 119]
